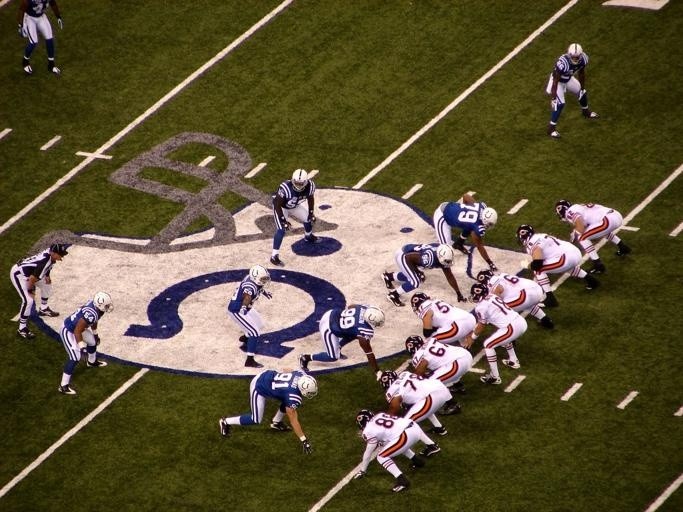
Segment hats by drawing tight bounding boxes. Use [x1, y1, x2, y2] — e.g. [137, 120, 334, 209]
[51, 244, 68, 256]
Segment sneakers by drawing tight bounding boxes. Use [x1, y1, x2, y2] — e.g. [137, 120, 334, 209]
[502, 359, 520, 369]
[409, 459, 425, 470]
[419, 443, 440, 456]
[449, 385, 466, 394]
[547, 123, 561, 137]
[299, 353, 310, 374]
[582, 109, 599, 118]
[426, 424, 447, 437]
[391, 482, 410, 492]
[480, 374, 501, 384]
[270, 420, 292, 431]
[240, 336, 248, 344]
[219, 417, 229, 437]
[382, 271, 395, 290]
[86, 359, 107, 367]
[39, 306, 59, 317]
[387, 289, 405, 306]
[245, 358, 263, 367]
[452, 242, 471, 254]
[615, 247, 632, 256]
[23, 58, 32, 74]
[58, 383, 76, 394]
[340, 353, 347, 359]
[537, 317, 553, 329]
[270, 254, 284, 267]
[585, 281, 600, 290]
[438, 404, 461, 414]
[17, 326, 36, 338]
[304, 234, 322, 244]
[589, 264, 607, 274]
[48, 61, 61, 73]
[538, 291, 558, 307]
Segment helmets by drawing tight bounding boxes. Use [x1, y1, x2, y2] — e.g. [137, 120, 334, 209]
[515, 224, 534, 247]
[554, 199, 572, 219]
[410, 292, 430, 314]
[380, 370, 397, 390]
[249, 264, 271, 288]
[568, 43, 583, 64]
[437, 243, 455, 266]
[468, 283, 489, 304]
[93, 291, 114, 313]
[364, 306, 386, 330]
[356, 409, 374, 431]
[481, 207, 497, 230]
[406, 335, 424, 357]
[477, 270, 494, 284]
[292, 168, 308, 190]
[298, 374, 318, 400]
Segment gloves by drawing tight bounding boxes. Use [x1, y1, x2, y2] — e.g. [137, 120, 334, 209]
[57, 17, 63, 30]
[354, 470, 366, 480]
[263, 290, 273, 299]
[415, 270, 425, 281]
[78, 348, 88, 357]
[490, 263, 497, 271]
[521, 258, 529, 270]
[577, 88, 587, 101]
[18, 23, 27, 38]
[240, 307, 247, 315]
[550, 98, 559, 112]
[279, 215, 291, 232]
[377, 369, 382, 384]
[458, 291, 467, 302]
[302, 439, 312, 454]
[94, 334, 100, 344]
[307, 209, 316, 223]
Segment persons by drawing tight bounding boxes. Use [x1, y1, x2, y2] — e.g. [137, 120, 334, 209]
[462, 282, 528, 385]
[546, 42, 601, 138]
[17, 0, 64, 74]
[476, 269, 554, 329]
[218, 369, 320, 455]
[378, 369, 453, 437]
[227, 265, 273, 369]
[355, 408, 426, 494]
[410, 291, 477, 348]
[404, 334, 473, 388]
[515, 224, 598, 309]
[57, 291, 112, 395]
[554, 199, 632, 275]
[10, 243, 69, 340]
[381, 241, 468, 308]
[432, 193, 498, 272]
[270, 168, 323, 268]
[298, 304, 386, 382]
